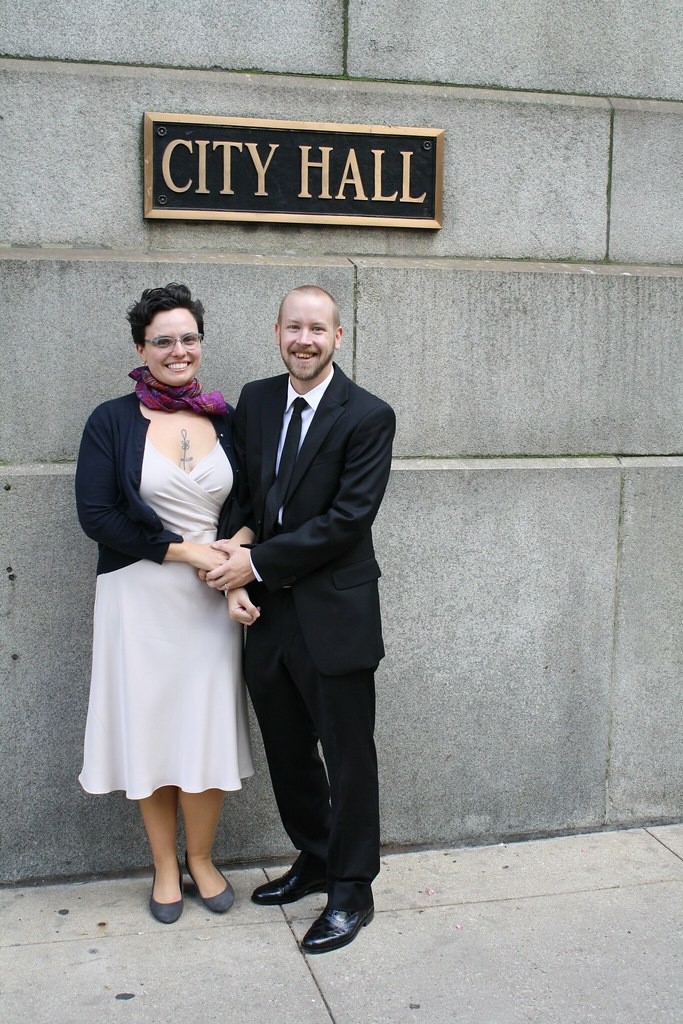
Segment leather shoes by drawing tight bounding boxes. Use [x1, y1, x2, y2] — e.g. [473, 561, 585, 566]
[251, 868, 331, 904]
[301, 904, 374, 955]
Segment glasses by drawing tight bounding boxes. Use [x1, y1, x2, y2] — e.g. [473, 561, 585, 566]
[144, 332, 203, 354]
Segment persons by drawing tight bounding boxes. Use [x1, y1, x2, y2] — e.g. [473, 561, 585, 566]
[76, 280, 262, 927]
[218, 283, 396, 955]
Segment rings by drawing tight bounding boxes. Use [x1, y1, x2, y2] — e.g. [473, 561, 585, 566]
[224, 583, 231, 590]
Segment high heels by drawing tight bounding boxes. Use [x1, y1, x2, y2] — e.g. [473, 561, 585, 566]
[150, 857, 183, 924]
[184, 852, 235, 913]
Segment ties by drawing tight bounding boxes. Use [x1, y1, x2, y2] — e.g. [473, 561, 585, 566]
[262, 397, 308, 542]
